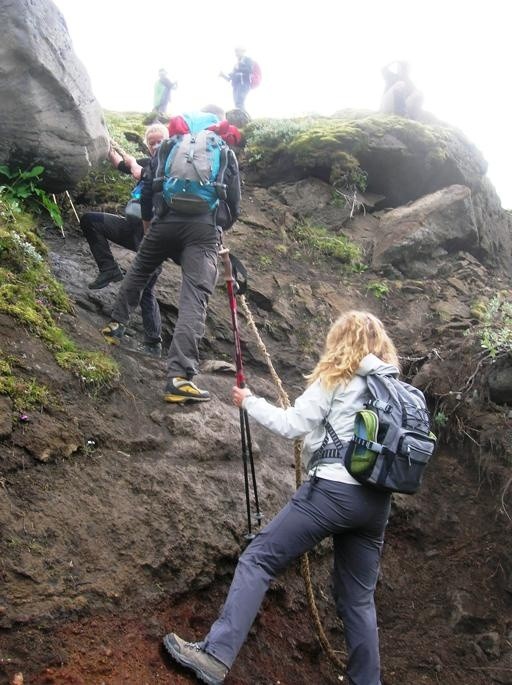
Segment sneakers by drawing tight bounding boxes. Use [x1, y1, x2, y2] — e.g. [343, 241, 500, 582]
[134, 342, 162, 360]
[88, 267, 123, 288]
[164, 377, 210, 402]
[164, 632, 229, 683]
[100, 319, 126, 346]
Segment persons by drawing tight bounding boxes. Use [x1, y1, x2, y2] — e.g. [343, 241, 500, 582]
[152, 67, 174, 116]
[163, 308, 400, 685]
[100, 103, 242, 405]
[219, 46, 253, 112]
[79, 122, 171, 358]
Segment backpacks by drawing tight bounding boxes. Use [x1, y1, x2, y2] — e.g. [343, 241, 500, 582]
[248, 60, 262, 88]
[309, 367, 437, 494]
[158, 130, 228, 216]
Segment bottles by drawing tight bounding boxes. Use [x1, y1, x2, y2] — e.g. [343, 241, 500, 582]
[130, 179, 144, 201]
